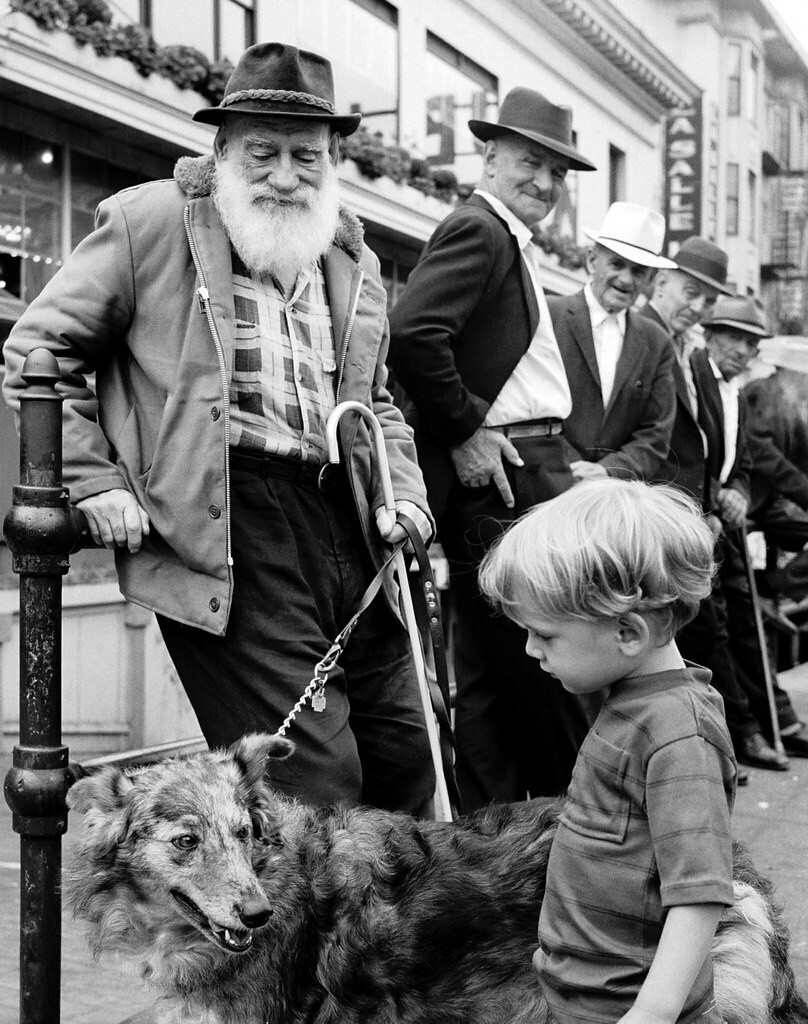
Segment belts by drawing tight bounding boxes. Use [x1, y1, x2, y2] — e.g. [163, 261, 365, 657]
[488, 424, 563, 439]
[228, 452, 352, 494]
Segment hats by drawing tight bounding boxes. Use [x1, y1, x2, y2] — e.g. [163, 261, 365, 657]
[700, 296, 773, 338]
[758, 335, 808, 374]
[192, 43, 361, 136]
[580, 201, 678, 268]
[469, 94, 596, 170]
[665, 238, 734, 299]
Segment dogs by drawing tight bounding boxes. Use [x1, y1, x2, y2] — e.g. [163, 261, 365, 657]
[54, 729, 808, 1024]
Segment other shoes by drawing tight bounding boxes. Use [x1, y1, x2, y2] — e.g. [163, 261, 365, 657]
[735, 770, 748, 785]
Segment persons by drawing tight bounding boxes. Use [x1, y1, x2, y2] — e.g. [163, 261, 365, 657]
[387, 86, 610, 814]
[635, 235, 791, 785]
[689, 294, 807, 739]
[543, 201, 677, 485]
[3, 43, 436, 817]
[477, 476, 738, 1024]
[739, 338, 808, 677]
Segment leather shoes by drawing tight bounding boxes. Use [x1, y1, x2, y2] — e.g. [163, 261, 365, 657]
[732, 734, 791, 771]
[765, 721, 808, 757]
